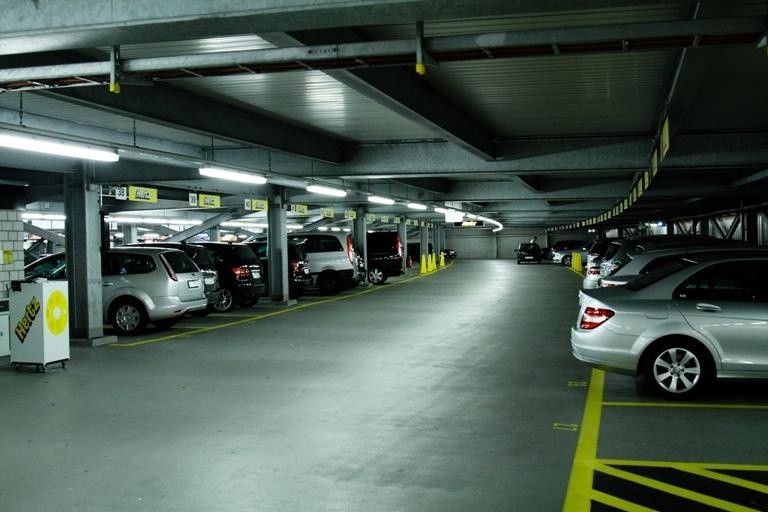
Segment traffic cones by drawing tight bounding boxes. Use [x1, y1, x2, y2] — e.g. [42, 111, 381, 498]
[427, 253, 437, 271]
[420, 255, 427, 274]
[440, 252, 446, 267]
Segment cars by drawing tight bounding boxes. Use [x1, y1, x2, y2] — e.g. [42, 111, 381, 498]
[440, 249, 456, 262]
[514, 243, 542, 264]
[552, 236, 768, 400]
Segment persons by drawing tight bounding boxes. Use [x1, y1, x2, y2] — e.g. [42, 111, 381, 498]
[529, 236, 538, 249]
